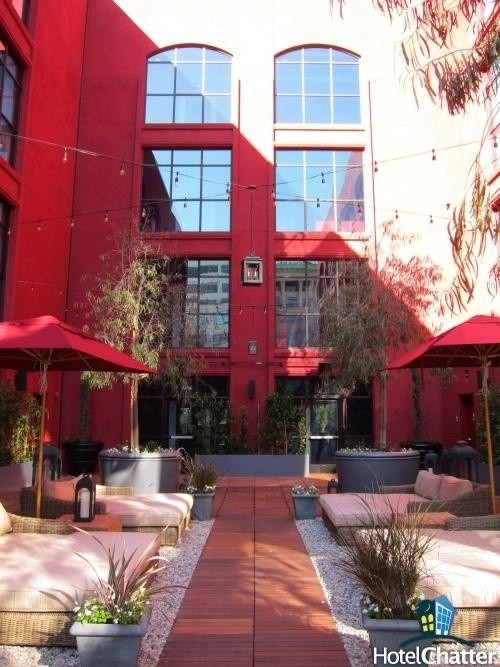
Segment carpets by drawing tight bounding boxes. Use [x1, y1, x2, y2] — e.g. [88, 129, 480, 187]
[0, 518, 216, 666]
[293, 517, 500, 666]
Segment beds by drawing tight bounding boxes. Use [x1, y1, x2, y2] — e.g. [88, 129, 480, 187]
[21, 475, 193, 545]
[0, 503, 157, 646]
[317, 470, 492, 545]
[356, 516, 500, 643]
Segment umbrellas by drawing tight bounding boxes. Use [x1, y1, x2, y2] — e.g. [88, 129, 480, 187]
[1, 315, 159, 518]
[378, 314, 499, 514]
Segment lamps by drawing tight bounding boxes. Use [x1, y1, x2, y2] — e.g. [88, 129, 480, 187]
[240, 188, 264, 286]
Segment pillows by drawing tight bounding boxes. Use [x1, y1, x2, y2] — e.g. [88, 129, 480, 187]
[46, 480, 74, 500]
[54, 487, 75, 501]
[0, 501, 13, 537]
[437, 475, 473, 502]
[414, 470, 442, 501]
[74, 473, 84, 488]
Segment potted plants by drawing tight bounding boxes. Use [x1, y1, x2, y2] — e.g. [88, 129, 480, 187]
[64, 523, 191, 667]
[181, 456, 223, 521]
[333, 458, 462, 667]
[290, 482, 319, 522]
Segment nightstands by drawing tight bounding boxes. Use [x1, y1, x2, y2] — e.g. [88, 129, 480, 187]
[56, 512, 125, 531]
